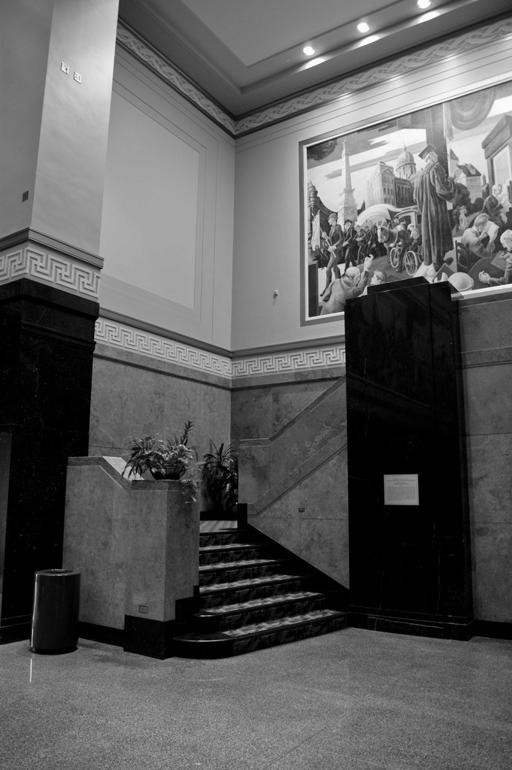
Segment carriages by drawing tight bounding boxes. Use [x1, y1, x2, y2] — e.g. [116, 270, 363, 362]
[376, 205, 422, 264]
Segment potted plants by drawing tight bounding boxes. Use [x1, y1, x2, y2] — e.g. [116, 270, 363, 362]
[120, 419, 239, 514]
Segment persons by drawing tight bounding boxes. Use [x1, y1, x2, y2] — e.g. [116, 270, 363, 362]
[308, 142, 512, 316]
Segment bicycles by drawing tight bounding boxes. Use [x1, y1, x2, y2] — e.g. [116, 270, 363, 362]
[390, 241, 424, 277]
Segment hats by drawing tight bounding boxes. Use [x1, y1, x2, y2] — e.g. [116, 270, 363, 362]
[417, 144, 435, 160]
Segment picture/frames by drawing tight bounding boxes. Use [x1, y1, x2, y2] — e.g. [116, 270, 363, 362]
[296, 73, 512, 329]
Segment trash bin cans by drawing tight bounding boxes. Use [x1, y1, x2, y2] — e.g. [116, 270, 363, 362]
[30, 569, 81, 655]
